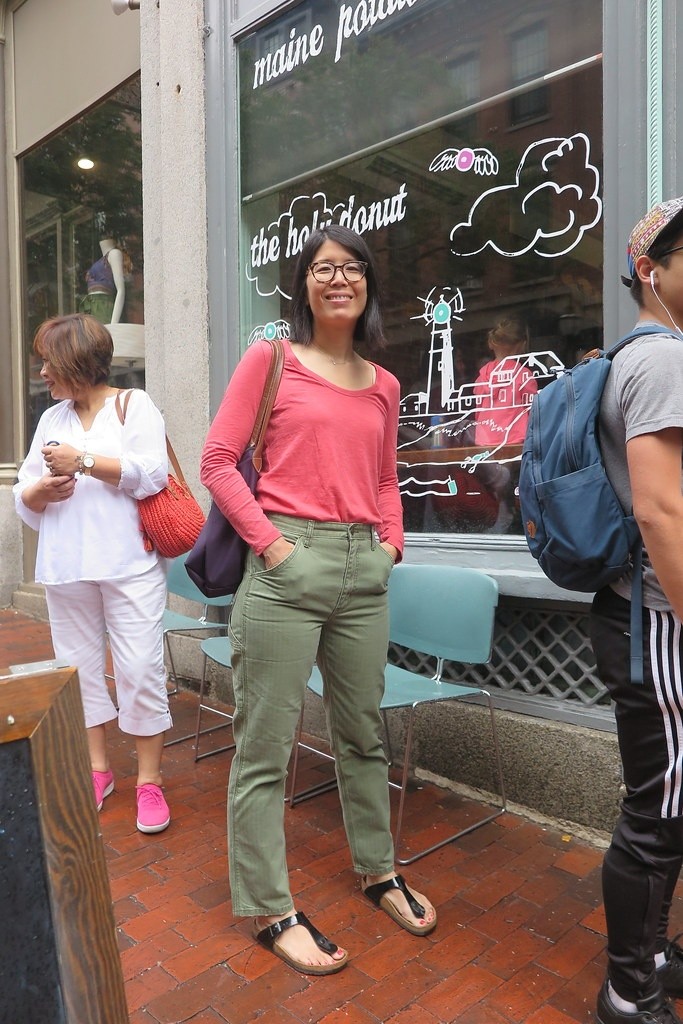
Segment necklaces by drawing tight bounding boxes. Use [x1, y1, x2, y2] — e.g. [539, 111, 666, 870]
[331, 352, 356, 365]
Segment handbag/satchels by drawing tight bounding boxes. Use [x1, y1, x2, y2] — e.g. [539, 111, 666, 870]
[116, 389, 206, 558]
[185, 339, 284, 598]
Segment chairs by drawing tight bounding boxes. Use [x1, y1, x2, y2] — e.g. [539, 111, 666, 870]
[104, 553, 235, 750]
[192, 635, 336, 804]
[291, 563, 508, 867]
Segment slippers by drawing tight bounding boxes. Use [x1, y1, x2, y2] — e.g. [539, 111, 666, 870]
[253, 913, 349, 977]
[361, 873, 437, 937]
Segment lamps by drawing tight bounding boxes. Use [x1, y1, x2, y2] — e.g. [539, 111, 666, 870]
[111, 0, 140, 17]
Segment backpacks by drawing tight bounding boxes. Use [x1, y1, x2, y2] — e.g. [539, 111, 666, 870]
[519, 327, 683, 592]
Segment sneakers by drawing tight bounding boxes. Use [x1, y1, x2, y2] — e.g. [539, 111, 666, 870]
[92, 769, 114, 811]
[137, 783, 169, 834]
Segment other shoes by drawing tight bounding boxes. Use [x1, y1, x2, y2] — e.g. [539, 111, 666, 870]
[596, 940, 683, 1024]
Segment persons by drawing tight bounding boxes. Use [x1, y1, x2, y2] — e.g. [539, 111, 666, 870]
[12, 314, 173, 833]
[200, 225, 438, 975]
[78, 238, 126, 326]
[420, 337, 514, 533]
[473, 315, 537, 446]
[587, 196, 683, 1024]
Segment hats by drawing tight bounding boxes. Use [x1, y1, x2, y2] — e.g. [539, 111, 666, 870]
[626, 196, 683, 279]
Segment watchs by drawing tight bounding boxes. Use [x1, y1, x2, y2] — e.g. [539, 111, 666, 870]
[83, 455, 95, 476]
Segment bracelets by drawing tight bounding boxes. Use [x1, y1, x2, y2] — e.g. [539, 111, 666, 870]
[79, 453, 83, 475]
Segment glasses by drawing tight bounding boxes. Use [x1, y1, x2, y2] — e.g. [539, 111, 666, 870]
[305, 261, 369, 283]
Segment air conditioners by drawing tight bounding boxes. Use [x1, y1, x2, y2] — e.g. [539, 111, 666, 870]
[337, 124, 497, 225]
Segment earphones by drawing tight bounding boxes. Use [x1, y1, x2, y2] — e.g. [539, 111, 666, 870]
[650, 270, 656, 284]
